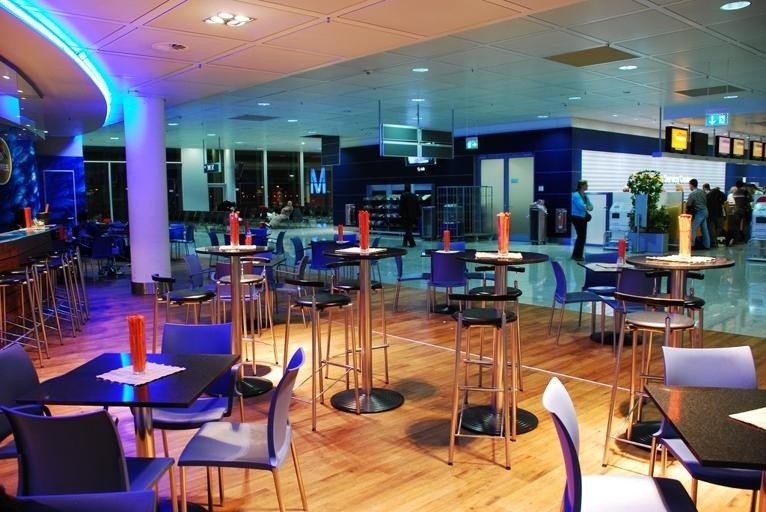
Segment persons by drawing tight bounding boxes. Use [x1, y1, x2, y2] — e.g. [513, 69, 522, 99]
[686, 179, 766, 249]
[264, 200, 293, 228]
[570, 180, 593, 261]
[217, 201, 243, 218]
[398, 184, 420, 246]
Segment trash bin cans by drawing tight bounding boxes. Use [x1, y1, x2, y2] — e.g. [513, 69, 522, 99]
[345, 204, 357, 226]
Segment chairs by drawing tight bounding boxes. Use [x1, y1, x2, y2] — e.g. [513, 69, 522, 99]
[541, 254, 766, 510]
[0, 314, 307, 510]
[1, 208, 705, 436]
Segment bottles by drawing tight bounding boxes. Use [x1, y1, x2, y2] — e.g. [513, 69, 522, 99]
[36, 221, 44, 228]
[245, 230, 251, 245]
[678, 214, 693, 260]
[617, 239, 626, 266]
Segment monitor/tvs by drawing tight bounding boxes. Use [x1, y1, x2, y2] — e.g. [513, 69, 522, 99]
[691, 131, 708, 156]
[404, 157, 437, 167]
[751, 140, 764, 160]
[717, 136, 732, 157]
[666, 126, 689, 153]
[733, 137, 745, 158]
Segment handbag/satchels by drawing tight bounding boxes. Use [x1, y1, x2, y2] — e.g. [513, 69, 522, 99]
[585, 213, 591, 222]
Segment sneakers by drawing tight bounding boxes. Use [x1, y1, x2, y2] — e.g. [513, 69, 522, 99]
[571, 256, 584, 261]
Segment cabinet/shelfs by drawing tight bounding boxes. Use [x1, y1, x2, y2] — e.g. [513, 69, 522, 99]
[362, 193, 431, 236]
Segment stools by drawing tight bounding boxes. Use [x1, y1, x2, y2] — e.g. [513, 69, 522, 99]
[448, 293, 518, 470]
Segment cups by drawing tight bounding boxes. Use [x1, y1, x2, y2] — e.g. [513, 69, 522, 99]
[259, 222, 264, 229]
[497, 213, 509, 256]
[359, 212, 369, 255]
[338, 225, 343, 243]
[127, 315, 146, 376]
[230, 213, 239, 249]
[443, 231, 451, 252]
[24, 207, 31, 228]
